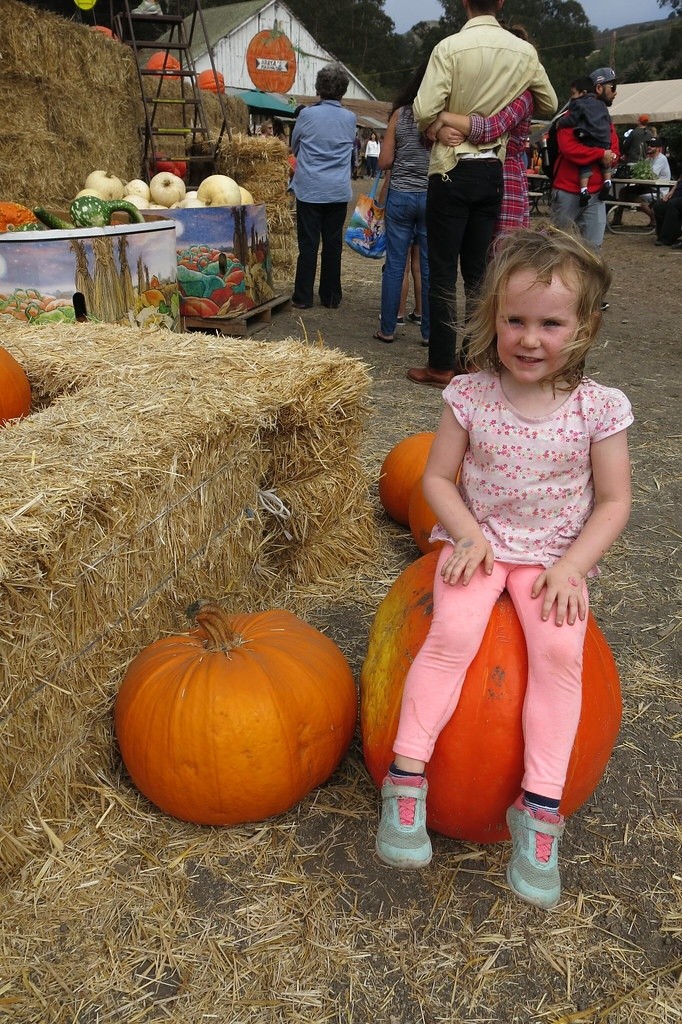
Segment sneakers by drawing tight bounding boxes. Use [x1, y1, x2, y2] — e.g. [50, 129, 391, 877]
[579, 189, 591, 207]
[599, 181, 611, 201]
[379, 314, 406, 325]
[505, 792, 566, 909]
[406, 309, 422, 325]
[376, 774, 431, 870]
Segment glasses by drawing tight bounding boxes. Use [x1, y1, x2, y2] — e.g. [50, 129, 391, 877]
[648, 143, 656, 147]
[602, 84, 616, 93]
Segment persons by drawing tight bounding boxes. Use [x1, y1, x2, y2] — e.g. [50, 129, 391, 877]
[285, 65, 356, 308]
[608, 114, 682, 247]
[375, 224, 635, 909]
[259, 104, 385, 179]
[373, 0, 620, 389]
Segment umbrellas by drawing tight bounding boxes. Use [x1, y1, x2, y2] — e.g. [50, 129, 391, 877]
[231, 89, 295, 117]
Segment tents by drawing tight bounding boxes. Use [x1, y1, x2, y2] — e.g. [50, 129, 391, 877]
[552, 79, 682, 124]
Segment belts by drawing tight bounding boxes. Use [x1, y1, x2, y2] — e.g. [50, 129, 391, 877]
[459, 158, 499, 162]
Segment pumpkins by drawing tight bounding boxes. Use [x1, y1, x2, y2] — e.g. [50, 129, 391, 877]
[360, 548, 623, 843]
[197, 70, 226, 95]
[0, 345, 31, 425]
[91, 25, 119, 41]
[115, 595, 357, 824]
[147, 51, 181, 80]
[0, 169, 253, 235]
[525, 165, 541, 174]
[380, 431, 458, 528]
[408, 471, 452, 553]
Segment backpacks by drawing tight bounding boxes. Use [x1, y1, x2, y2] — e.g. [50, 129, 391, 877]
[535, 110, 568, 178]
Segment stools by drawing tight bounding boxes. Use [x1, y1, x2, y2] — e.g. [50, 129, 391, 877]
[529, 192, 542, 213]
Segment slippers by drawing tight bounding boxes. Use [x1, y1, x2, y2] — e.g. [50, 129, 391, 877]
[373, 331, 394, 343]
[421, 341, 428, 347]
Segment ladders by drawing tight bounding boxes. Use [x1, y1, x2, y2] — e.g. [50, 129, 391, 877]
[108, 1, 219, 191]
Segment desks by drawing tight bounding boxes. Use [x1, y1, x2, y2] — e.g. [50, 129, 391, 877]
[601, 180, 676, 233]
[528, 175, 548, 214]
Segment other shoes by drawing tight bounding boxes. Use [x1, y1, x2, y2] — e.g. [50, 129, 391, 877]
[620, 156, 626, 162]
[655, 239, 668, 245]
[325, 304, 339, 309]
[644, 217, 656, 229]
[609, 223, 624, 227]
[291, 300, 308, 308]
[666, 230, 682, 245]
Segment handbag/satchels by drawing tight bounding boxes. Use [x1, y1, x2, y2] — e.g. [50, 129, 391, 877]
[613, 164, 631, 179]
[344, 169, 391, 259]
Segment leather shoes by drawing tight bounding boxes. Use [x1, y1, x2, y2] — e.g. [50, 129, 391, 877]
[456, 363, 481, 374]
[408, 365, 454, 389]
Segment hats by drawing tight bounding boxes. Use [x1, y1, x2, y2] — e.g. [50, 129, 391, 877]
[588, 67, 616, 86]
[645, 137, 663, 145]
[638, 115, 648, 122]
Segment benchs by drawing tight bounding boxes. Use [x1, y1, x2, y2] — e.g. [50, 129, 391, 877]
[605, 201, 641, 232]
